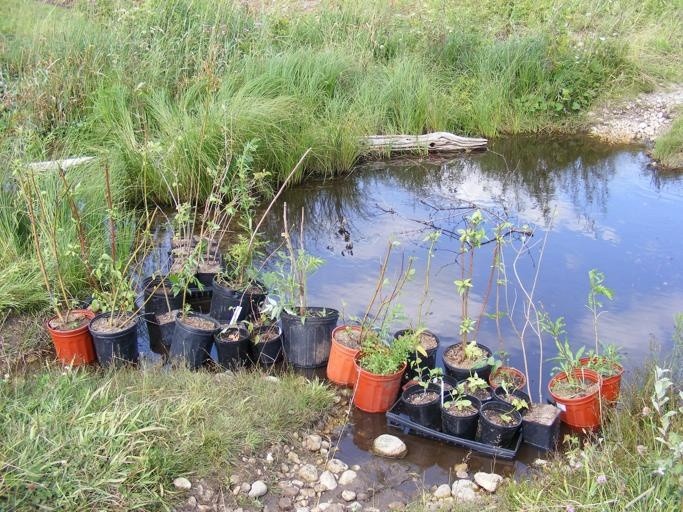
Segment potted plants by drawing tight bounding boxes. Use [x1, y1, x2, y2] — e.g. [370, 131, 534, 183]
[326, 243, 414, 385]
[535, 299, 605, 434]
[54, 161, 142, 373]
[480, 211, 522, 450]
[491, 210, 531, 415]
[440, 228, 481, 438]
[400, 316, 441, 430]
[81, 162, 143, 318]
[142, 74, 277, 353]
[426, 367, 456, 393]
[453, 225, 494, 402]
[346, 258, 427, 413]
[215, 221, 296, 370]
[249, 291, 281, 367]
[442, 208, 492, 382]
[14, 167, 95, 366]
[574, 264, 625, 408]
[167, 237, 219, 371]
[395, 229, 443, 380]
[509, 204, 562, 452]
[488, 210, 528, 392]
[262, 200, 339, 370]
